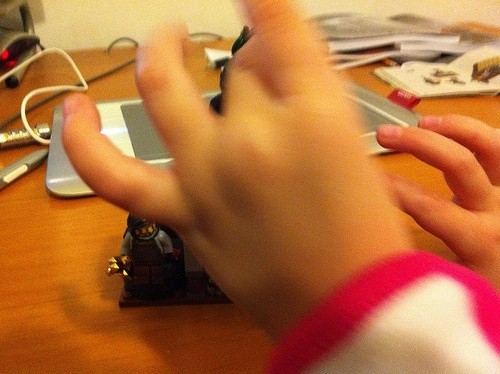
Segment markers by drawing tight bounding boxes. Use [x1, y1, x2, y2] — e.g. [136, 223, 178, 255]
[5, 49, 36, 88]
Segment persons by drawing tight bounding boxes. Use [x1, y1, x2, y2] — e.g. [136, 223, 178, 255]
[63, 0, 499, 374]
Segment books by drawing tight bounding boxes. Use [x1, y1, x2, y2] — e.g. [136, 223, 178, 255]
[311, 9, 460, 55]
[388, 13, 500, 57]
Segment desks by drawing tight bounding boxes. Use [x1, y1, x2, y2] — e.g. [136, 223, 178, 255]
[0, 37, 498, 374]
